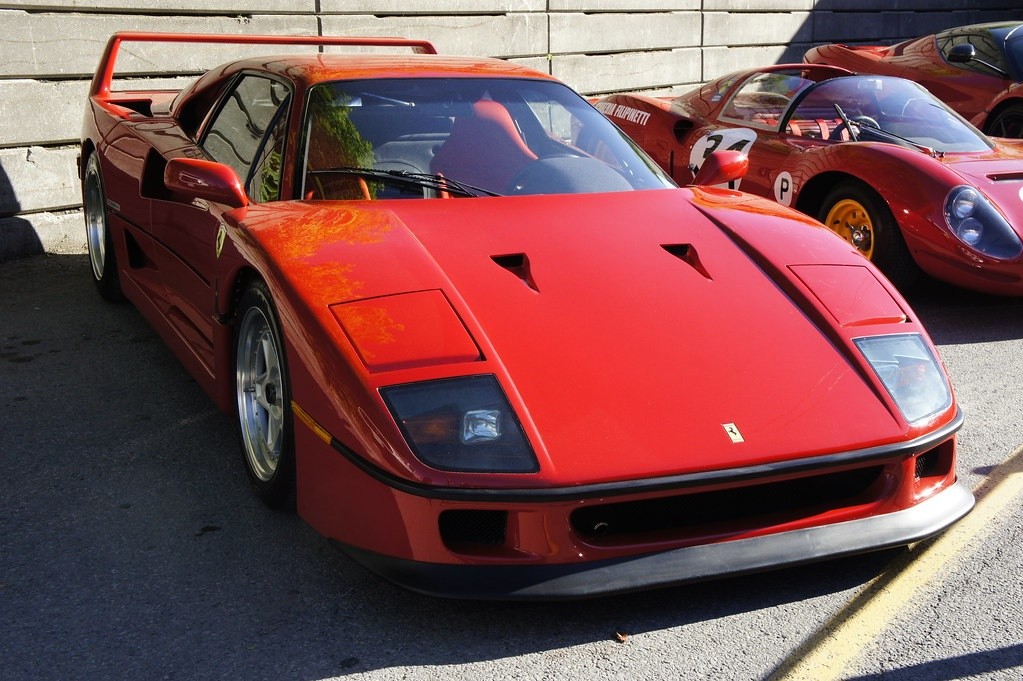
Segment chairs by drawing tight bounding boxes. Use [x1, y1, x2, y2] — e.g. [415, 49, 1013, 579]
[244, 106, 372, 200]
[430, 99, 541, 198]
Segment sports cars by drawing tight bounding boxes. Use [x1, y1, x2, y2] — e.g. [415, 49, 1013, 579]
[77, 31, 977, 602]
[784, 21, 1023, 139]
[571, 64, 1023, 299]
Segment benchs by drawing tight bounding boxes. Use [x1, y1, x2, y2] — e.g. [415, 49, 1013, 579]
[751, 117, 861, 141]
